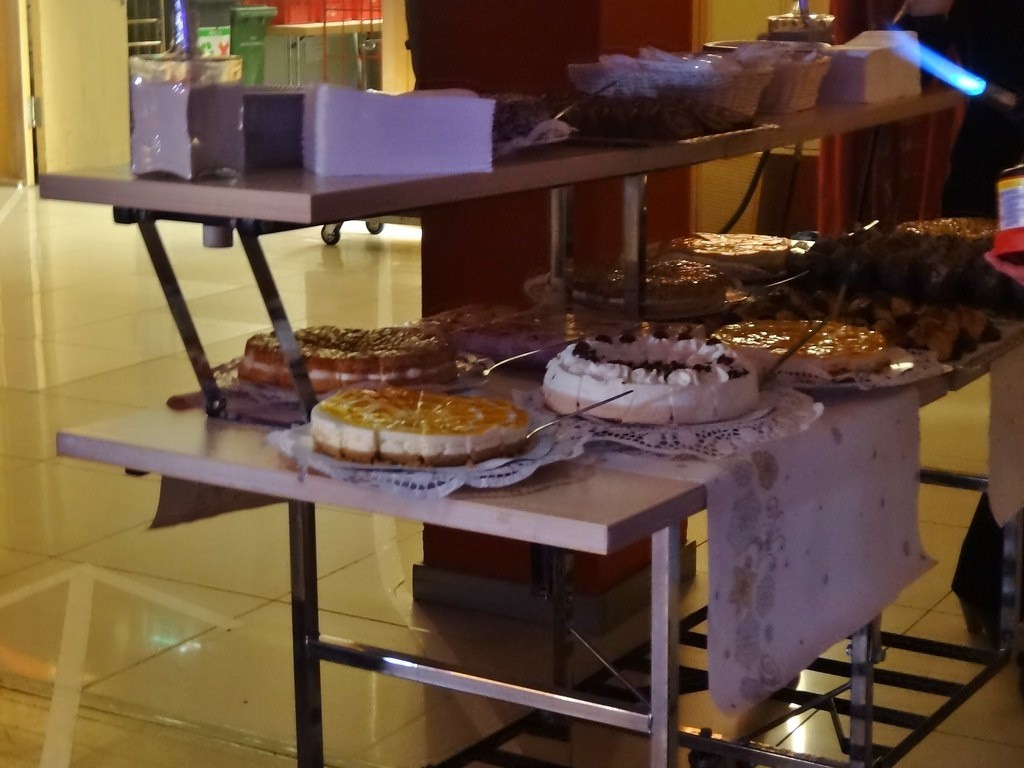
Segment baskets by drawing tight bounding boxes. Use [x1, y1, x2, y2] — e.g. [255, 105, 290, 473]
[567, 60, 777, 134]
[702, 39, 832, 116]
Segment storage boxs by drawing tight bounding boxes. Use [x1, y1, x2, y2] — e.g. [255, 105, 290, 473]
[565, 58, 774, 137]
[128, 53, 246, 181]
[704, 38, 833, 117]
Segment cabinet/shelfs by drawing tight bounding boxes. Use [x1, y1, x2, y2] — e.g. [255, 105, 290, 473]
[33, 88, 1024, 768]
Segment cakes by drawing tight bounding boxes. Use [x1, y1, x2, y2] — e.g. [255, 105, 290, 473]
[241, 215, 997, 469]
